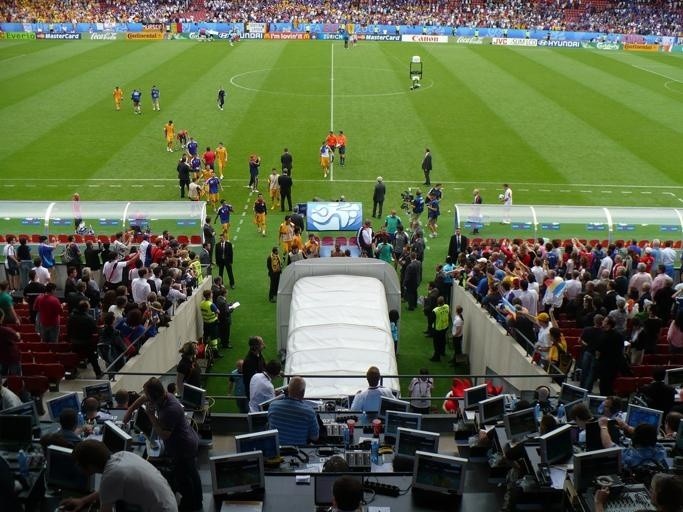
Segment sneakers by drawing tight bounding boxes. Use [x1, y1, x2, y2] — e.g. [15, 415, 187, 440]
[221, 345, 232, 349]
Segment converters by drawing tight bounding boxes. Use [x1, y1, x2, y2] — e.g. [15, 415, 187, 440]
[320, 447, 334, 454]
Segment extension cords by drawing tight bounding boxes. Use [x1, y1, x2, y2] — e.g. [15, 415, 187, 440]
[364, 480, 399, 497]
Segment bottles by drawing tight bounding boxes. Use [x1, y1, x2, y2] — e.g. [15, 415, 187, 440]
[77, 412, 84, 427]
[535, 403, 541, 419]
[371, 434, 380, 464]
[137, 431, 145, 442]
[317, 402, 325, 412]
[17, 450, 29, 478]
[556, 404, 564, 419]
[512, 397, 516, 406]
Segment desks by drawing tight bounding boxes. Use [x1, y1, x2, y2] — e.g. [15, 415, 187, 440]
[2, 399, 683, 508]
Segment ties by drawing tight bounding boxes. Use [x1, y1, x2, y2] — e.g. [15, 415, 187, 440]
[221, 244, 223, 255]
[458, 236, 460, 247]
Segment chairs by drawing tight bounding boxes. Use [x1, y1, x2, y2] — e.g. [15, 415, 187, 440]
[0, 233, 201, 403]
[308, 235, 360, 247]
[170, 0, 609, 33]
[462, 237, 681, 399]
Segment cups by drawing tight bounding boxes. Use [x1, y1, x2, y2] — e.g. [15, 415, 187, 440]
[347, 419, 355, 436]
[373, 419, 381, 437]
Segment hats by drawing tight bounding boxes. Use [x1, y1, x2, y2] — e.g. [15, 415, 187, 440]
[534, 313, 549, 322]
[477, 258, 487, 263]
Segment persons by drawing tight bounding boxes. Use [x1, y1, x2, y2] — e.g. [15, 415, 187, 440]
[217, 87, 225, 110]
[113, 87, 124, 111]
[131, 90, 141, 115]
[410, 74, 421, 90]
[151, 86, 160, 111]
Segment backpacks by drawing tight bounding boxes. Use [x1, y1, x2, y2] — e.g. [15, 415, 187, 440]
[63, 243, 76, 263]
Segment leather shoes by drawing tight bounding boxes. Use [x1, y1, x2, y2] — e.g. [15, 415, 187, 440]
[231, 285, 235, 289]
[214, 355, 224, 359]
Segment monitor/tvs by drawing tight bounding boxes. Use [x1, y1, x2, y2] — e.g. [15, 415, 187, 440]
[413, 451, 468, 496]
[209, 450, 265, 496]
[248, 411, 269, 433]
[0, 382, 206, 512]
[348, 395, 356, 408]
[256, 482, 259, 483]
[464, 368, 683, 497]
[258, 394, 285, 411]
[395, 428, 440, 461]
[235, 429, 280, 459]
[275, 385, 288, 397]
[378, 396, 411, 421]
[384, 410, 422, 441]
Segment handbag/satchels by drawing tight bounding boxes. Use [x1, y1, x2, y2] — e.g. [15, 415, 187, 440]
[102, 281, 111, 292]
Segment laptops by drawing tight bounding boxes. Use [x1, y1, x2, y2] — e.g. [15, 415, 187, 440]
[316, 473, 364, 512]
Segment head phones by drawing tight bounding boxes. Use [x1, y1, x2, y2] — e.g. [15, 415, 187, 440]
[325, 401, 335, 410]
